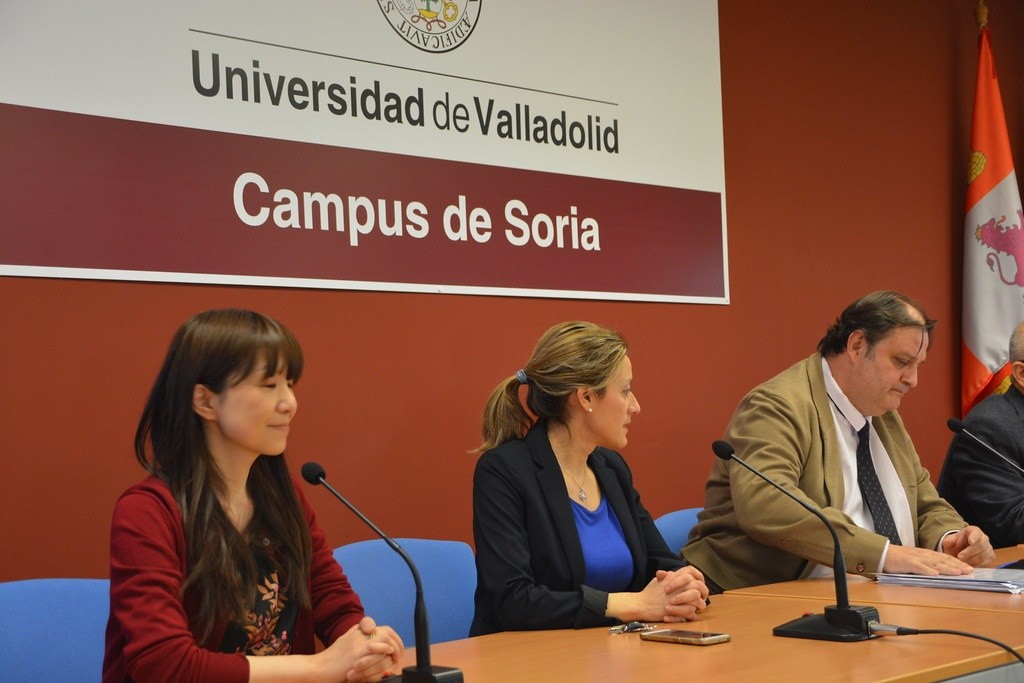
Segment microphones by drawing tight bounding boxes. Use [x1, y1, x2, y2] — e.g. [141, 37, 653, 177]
[711, 439, 882, 642]
[300, 460, 465, 683]
[947, 416, 1024, 477]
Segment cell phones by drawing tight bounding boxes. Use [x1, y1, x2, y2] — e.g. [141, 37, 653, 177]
[640, 629, 730, 646]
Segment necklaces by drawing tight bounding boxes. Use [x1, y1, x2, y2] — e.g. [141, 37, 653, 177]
[549, 438, 588, 502]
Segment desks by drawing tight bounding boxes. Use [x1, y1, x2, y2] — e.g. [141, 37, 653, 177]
[396, 593, 1024, 683]
[722, 544, 1024, 614]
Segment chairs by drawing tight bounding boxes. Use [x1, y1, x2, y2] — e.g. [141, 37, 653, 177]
[0, 578, 111, 683]
[653, 508, 704, 554]
[333, 537, 477, 646]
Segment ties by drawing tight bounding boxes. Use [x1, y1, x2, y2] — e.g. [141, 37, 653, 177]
[857, 420, 903, 547]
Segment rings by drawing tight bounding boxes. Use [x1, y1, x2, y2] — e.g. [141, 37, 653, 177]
[370, 632, 375, 639]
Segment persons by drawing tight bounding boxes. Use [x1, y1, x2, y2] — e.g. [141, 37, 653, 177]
[101, 308, 404, 683]
[678, 290, 995, 595]
[465, 320, 711, 638]
[937, 321, 1024, 550]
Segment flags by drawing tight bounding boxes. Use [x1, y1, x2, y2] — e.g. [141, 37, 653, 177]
[959, 23, 1024, 420]
